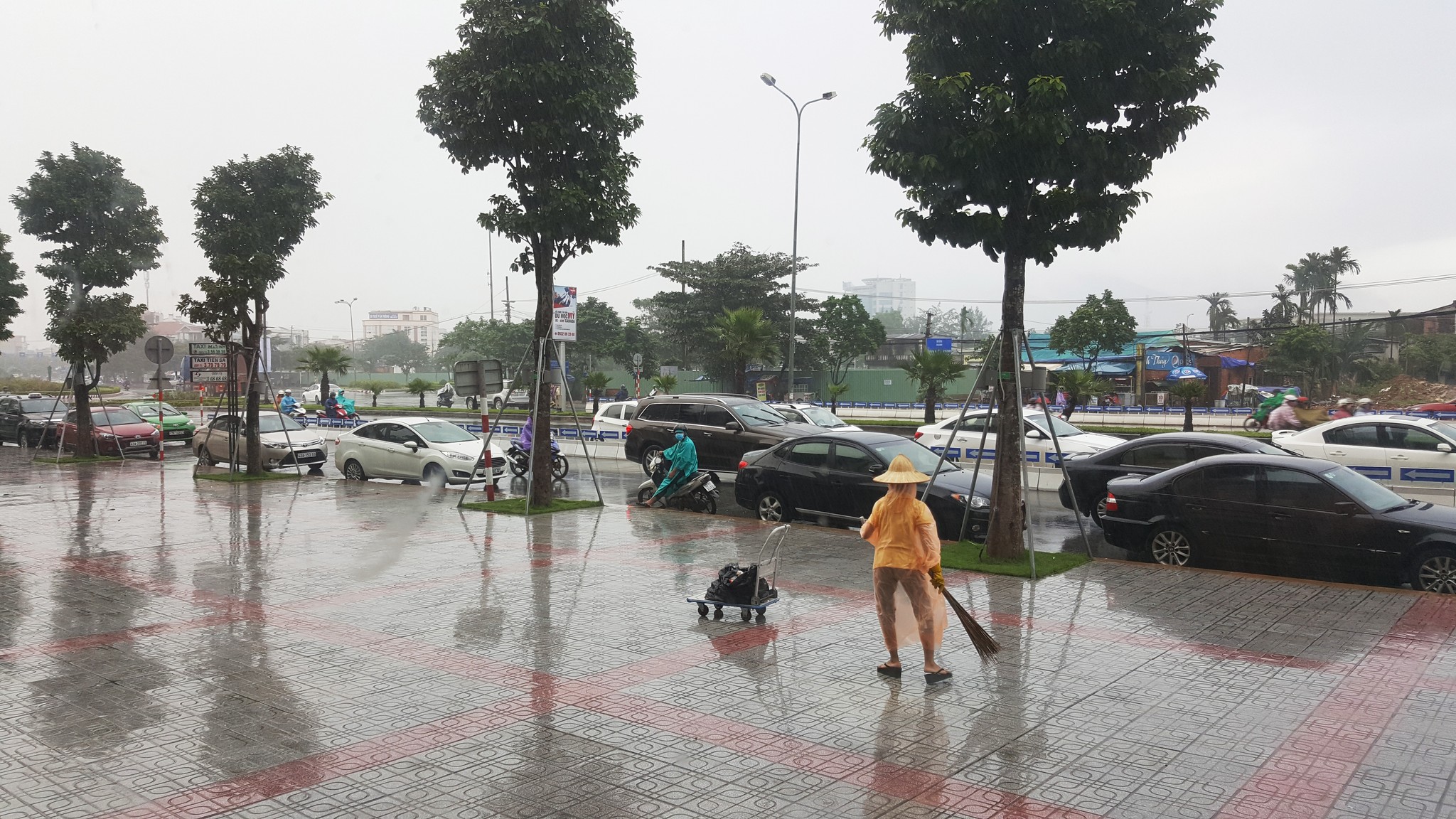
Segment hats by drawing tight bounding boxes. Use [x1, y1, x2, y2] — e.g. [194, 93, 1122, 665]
[338, 390, 344, 394]
[872, 454, 931, 484]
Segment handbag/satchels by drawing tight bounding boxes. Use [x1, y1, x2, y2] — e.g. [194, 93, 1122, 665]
[704, 561, 769, 605]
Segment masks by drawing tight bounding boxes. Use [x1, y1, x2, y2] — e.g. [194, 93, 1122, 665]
[675, 433, 684, 441]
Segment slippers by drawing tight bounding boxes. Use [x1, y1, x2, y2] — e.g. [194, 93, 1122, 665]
[636, 502, 651, 508]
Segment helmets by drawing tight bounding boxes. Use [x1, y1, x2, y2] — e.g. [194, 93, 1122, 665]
[2, 386, 8, 390]
[621, 384, 625, 388]
[1357, 398, 1375, 405]
[673, 423, 688, 435]
[284, 389, 291, 393]
[1283, 395, 1298, 402]
[551, 385, 555, 389]
[1035, 396, 1051, 404]
[1336, 397, 1355, 408]
[278, 390, 284, 394]
[529, 409, 534, 415]
[329, 392, 336, 396]
[1027, 397, 1037, 404]
[125, 377, 128, 379]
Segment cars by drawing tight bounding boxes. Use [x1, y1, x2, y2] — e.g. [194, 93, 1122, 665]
[913, 408, 1128, 471]
[121, 401, 195, 444]
[1100, 453, 1456, 597]
[1272, 412, 1454, 490]
[335, 414, 510, 490]
[303, 384, 343, 403]
[192, 412, 328, 473]
[1059, 431, 1300, 531]
[733, 430, 1031, 545]
[591, 400, 637, 433]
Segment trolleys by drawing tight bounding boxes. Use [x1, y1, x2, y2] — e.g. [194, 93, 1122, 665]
[687, 523, 790, 621]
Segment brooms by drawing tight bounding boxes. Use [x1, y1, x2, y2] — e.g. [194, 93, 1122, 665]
[924, 566, 1008, 666]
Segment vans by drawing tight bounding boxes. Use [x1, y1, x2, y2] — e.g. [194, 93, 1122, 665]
[168, 373, 179, 385]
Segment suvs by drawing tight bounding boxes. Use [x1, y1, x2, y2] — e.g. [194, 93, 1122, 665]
[621, 392, 832, 476]
[1, 393, 69, 450]
[58, 405, 160, 459]
[757, 398, 863, 433]
[467, 379, 531, 411]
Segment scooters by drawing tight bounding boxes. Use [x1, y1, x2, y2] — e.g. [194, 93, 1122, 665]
[283, 401, 308, 417]
[314, 401, 349, 427]
[341, 399, 361, 422]
[123, 382, 130, 390]
[436, 393, 455, 409]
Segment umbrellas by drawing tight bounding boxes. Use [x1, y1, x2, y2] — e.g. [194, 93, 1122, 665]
[1165, 365, 1207, 383]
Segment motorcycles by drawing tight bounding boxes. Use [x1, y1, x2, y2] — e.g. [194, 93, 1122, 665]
[637, 452, 715, 516]
[509, 428, 569, 478]
[1245, 403, 1274, 432]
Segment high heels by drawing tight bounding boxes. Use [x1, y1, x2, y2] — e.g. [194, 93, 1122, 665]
[923, 668, 952, 684]
[877, 663, 902, 678]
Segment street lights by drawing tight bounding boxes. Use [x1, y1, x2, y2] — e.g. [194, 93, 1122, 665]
[335, 297, 359, 388]
[761, 65, 838, 401]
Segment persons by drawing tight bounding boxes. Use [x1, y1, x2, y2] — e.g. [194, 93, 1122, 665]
[335, 389, 355, 409]
[616, 384, 629, 401]
[439, 383, 454, 401]
[281, 389, 304, 415]
[634, 423, 699, 508]
[550, 385, 560, 401]
[0, 386, 11, 394]
[860, 453, 955, 685]
[325, 392, 344, 419]
[1025, 383, 1432, 448]
[273, 390, 285, 410]
[31, 369, 131, 390]
[521, 408, 535, 450]
[180, 374, 184, 382]
